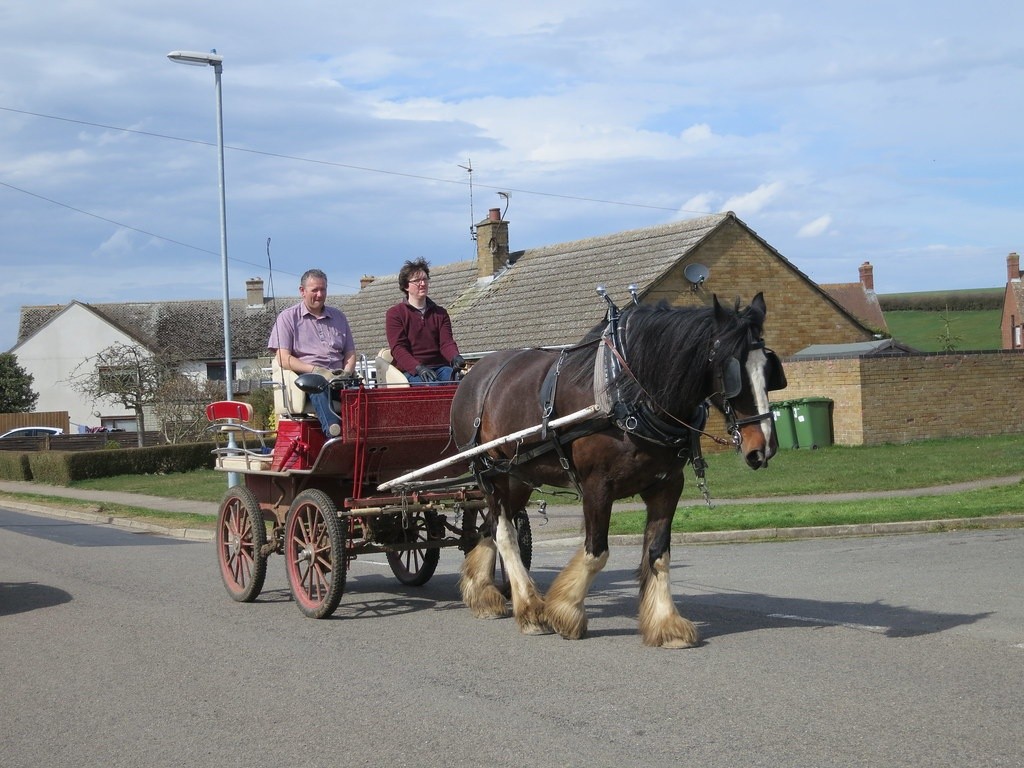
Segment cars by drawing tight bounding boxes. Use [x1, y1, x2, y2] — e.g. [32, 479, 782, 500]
[1, 426, 66, 438]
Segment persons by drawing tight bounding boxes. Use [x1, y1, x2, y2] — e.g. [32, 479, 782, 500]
[268, 269, 356, 441]
[386, 260, 465, 387]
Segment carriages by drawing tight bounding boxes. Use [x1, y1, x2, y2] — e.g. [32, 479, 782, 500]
[206, 284, 787, 650]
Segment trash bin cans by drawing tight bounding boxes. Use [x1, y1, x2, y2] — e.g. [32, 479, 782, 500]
[769, 399, 799, 450]
[791, 395, 833, 450]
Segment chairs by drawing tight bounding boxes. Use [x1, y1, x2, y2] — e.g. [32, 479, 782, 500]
[273, 357, 342, 417]
[204, 400, 277, 468]
[375, 348, 411, 387]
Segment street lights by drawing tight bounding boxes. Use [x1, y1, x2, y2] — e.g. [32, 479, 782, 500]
[166, 47, 242, 491]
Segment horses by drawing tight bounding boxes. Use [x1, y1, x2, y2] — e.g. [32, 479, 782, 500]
[449, 291, 779, 651]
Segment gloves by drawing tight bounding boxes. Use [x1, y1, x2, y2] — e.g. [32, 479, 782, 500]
[337, 369, 353, 384]
[416, 364, 438, 382]
[450, 356, 465, 374]
[311, 365, 340, 388]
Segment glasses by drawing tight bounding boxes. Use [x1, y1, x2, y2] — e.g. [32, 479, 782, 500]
[407, 277, 431, 285]
